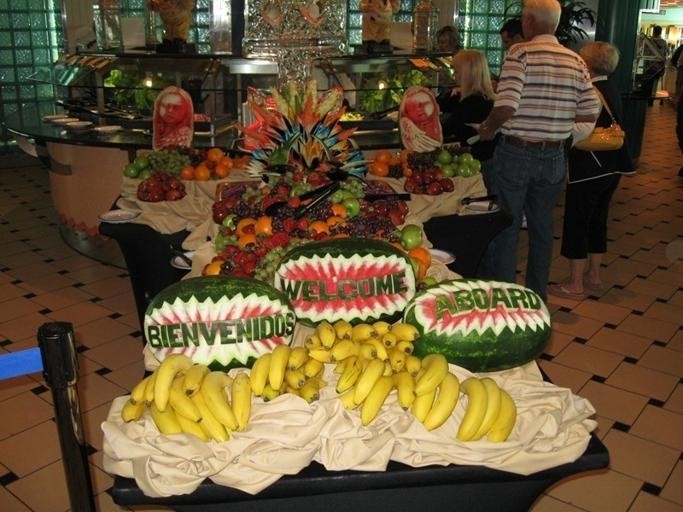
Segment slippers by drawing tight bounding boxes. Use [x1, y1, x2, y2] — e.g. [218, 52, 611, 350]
[549, 274, 604, 298]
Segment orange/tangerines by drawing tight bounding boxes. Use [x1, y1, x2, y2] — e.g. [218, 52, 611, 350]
[371, 148, 413, 177]
[181, 148, 233, 180]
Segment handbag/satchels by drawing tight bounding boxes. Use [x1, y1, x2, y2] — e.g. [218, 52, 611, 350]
[575, 125, 625, 152]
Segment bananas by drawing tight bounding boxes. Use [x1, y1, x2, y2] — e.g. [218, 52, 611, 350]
[121, 320, 517, 444]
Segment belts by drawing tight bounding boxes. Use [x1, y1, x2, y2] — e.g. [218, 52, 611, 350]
[504, 136, 564, 151]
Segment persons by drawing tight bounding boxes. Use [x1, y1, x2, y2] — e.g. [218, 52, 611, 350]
[153, 85, 192, 149]
[500, 18, 525, 48]
[643, 26, 667, 108]
[477, 0, 603, 305]
[443, 50, 497, 144]
[662, 44, 683, 175]
[548, 42, 637, 294]
[436, 25, 464, 53]
[401, 86, 441, 150]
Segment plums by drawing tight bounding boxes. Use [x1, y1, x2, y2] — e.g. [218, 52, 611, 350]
[404, 149, 481, 195]
[123, 156, 186, 202]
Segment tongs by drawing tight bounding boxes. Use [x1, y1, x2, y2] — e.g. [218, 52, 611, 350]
[167, 244, 192, 267]
[265, 181, 340, 222]
[460, 192, 498, 209]
[364, 193, 413, 203]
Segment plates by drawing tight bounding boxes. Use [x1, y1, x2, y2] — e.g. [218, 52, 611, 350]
[94, 209, 140, 223]
[172, 252, 194, 272]
[43, 114, 123, 135]
[464, 201, 496, 211]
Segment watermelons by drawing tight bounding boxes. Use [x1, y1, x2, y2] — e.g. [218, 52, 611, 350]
[401, 278, 551, 372]
[273, 237, 417, 328]
[142, 276, 296, 373]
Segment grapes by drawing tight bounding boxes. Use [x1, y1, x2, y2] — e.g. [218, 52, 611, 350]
[147, 144, 206, 180]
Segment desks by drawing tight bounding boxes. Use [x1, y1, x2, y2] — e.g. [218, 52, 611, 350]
[95, 177, 522, 373]
[108, 347, 611, 511]
